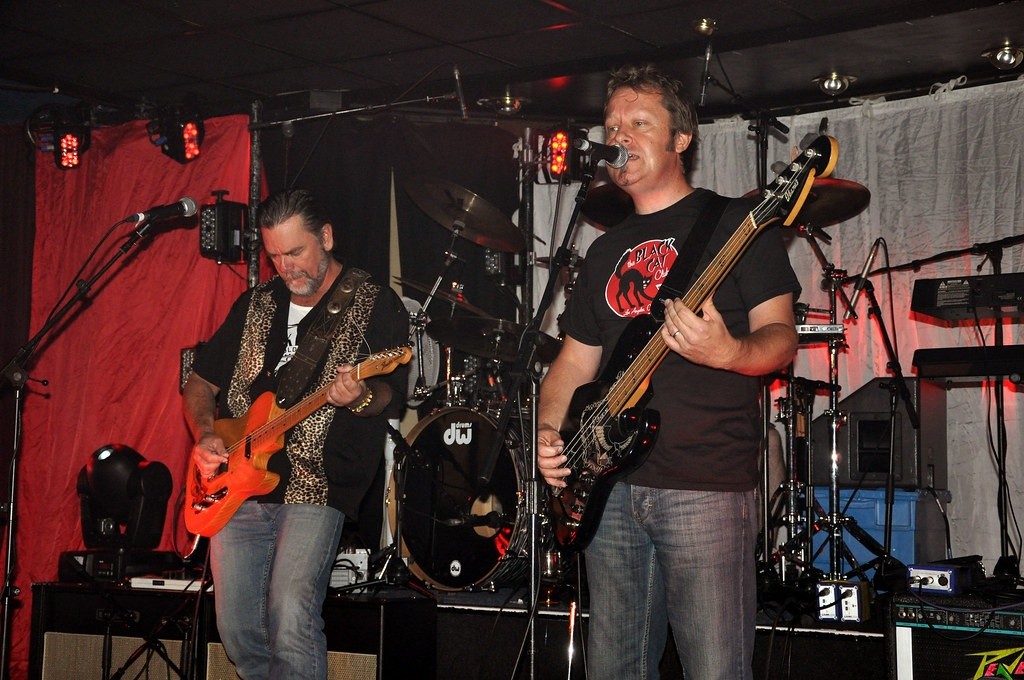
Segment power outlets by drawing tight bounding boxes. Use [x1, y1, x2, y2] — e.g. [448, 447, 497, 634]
[816, 579, 871, 624]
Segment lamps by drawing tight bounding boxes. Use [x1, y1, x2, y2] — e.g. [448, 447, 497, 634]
[980, 41, 1024, 70]
[145, 96, 206, 163]
[484, 247, 516, 286]
[199, 190, 248, 264]
[464, 356, 485, 396]
[477, 85, 530, 117]
[23, 102, 92, 171]
[540, 130, 582, 191]
[811, 72, 857, 95]
[177, 340, 207, 396]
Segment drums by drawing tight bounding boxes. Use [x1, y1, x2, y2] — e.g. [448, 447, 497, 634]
[386, 400, 556, 593]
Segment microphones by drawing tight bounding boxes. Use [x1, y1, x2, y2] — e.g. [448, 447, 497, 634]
[842, 238, 881, 319]
[699, 43, 712, 106]
[126, 197, 199, 223]
[570, 137, 628, 167]
[447, 511, 506, 528]
[453, 64, 469, 120]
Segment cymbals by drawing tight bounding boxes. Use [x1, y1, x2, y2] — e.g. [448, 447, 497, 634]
[579, 184, 635, 231]
[403, 174, 527, 254]
[741, 177, 871, 229]
[424, 318, 565, 367]
[392, 275, 493, 318]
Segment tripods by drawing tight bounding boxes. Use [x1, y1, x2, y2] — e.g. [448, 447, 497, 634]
[759, 220, 910, 599]
[326, 422, 481, 604]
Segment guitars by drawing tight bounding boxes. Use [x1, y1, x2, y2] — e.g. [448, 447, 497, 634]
[552, 117, 839, 553]
[184, 346, 412, 538]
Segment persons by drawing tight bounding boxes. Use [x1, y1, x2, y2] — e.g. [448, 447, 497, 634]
[535, 65, 802, 679]
[180, 186, 411, 680]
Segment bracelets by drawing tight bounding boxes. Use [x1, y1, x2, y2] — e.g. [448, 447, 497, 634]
[347, 388, 373, 413]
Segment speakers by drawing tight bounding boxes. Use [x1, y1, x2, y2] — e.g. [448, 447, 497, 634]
[42, 631, 381, 680]
[807, 376, 949, 490]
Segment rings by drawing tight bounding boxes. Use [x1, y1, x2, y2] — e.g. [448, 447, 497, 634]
[672, 331, 680, 338]
